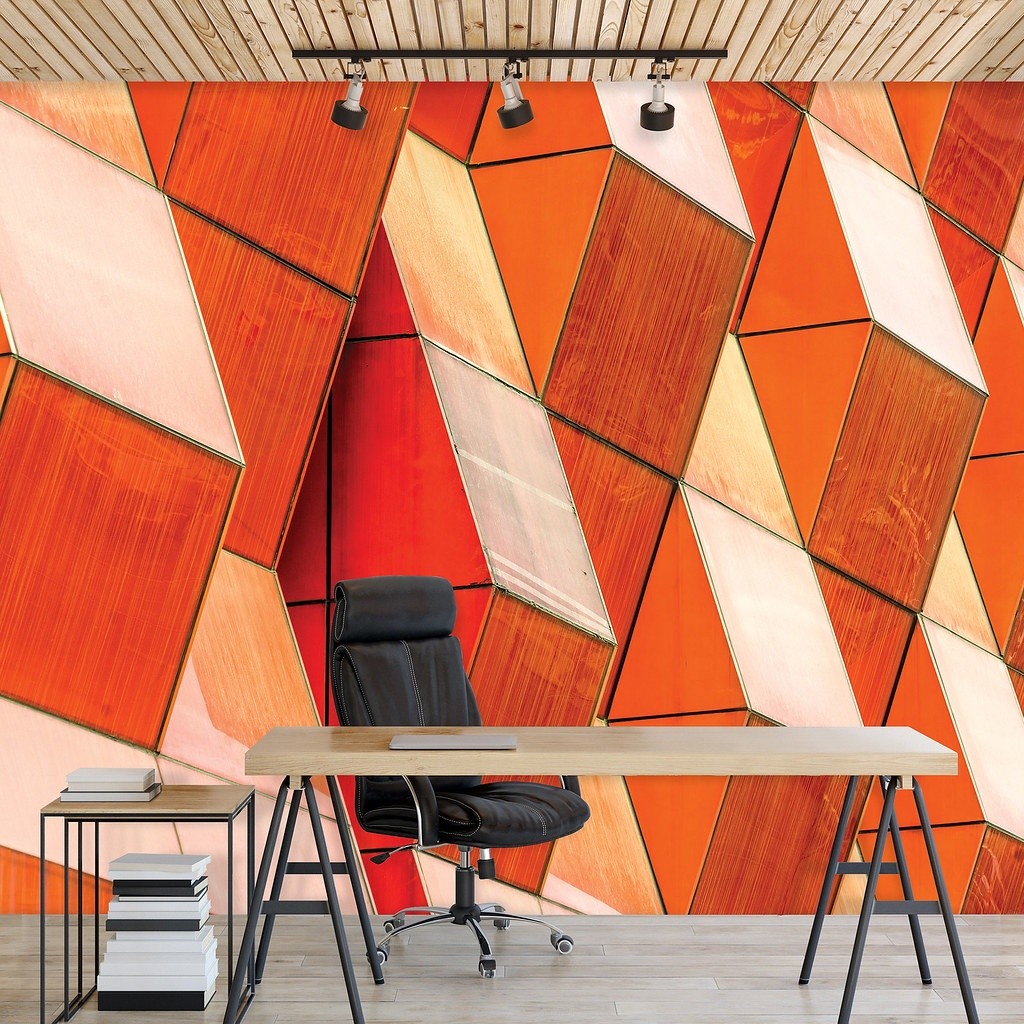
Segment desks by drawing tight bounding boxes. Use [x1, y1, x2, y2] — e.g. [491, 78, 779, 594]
[38, 785, 256, 1024]
[224, 726, 980, 1024]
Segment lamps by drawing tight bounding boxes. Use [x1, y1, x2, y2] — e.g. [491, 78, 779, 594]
[641, 59, 675, 132]
[332, 58, 368, 130]
[497, 58, 534, 129]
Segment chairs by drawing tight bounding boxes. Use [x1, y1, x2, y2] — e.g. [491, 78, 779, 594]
[331, 576, 590, 977]
